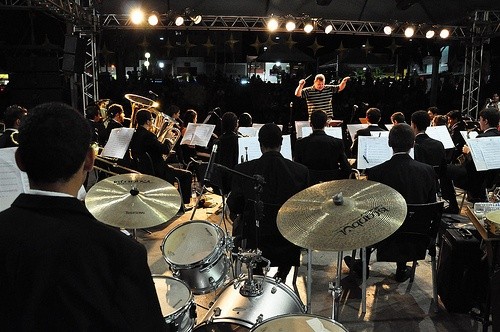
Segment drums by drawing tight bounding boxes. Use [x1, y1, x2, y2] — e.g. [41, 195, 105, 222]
[160, 220, 231, 295]
[191, 274, 305, 332]
[151, 273, 197, 332]
[249, 313, 349, 332]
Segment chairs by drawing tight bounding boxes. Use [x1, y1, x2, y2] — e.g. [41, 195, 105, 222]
[367, 161, 500, 285]
[96, 129, 302, 304]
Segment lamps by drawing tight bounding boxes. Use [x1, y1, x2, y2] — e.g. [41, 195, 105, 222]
[267, 12, 333, 35]
[383, 15, 452, 39]
[148, 7, 202, 26]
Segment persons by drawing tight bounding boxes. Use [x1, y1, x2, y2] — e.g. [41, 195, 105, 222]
[91, 62, 500, 127]
[294, 112, 352, 186]
[295, 74, 351, 121]
[344, 124, 441, 283]
[350, 92, 500, 215]
[0, 103, 239, 332]
[228, 126, 311, 286]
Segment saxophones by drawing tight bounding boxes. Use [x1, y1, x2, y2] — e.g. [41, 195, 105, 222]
[456, 125, 480, 165]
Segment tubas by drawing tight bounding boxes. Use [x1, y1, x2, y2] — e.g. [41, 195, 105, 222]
[123, 93, 181, 161]
[95, 99, 112, 128]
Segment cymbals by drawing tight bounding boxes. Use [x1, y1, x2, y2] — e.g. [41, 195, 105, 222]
[84, 173, 182, 229]
[276, 179, 408, 252]
